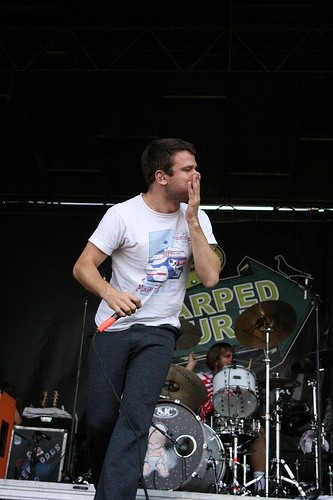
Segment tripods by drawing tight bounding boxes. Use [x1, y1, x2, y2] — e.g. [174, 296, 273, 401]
[219, 327, 307, 500]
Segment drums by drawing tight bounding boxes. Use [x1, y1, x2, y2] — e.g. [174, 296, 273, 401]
[138, 398, 224, 491]
[210, 364, 258, 418]
[210, 413, 259, 444]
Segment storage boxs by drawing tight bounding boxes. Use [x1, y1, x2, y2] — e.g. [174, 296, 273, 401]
[4, 424, 69, 482]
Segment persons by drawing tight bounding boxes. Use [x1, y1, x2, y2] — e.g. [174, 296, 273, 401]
[73, 138, 221, 500]
[0, 381, 22, 424]
[185, 341, 268, 496]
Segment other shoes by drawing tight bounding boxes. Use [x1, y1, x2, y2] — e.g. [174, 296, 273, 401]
[254, 489, 270, 497]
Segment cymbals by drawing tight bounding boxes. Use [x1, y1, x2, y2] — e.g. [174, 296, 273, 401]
[175, 316, 202, 351]
[292, 347, 332, 373]
[259, 375, 303, 390]
[233, 299, 298, 350]
[158, 364, 209, 411]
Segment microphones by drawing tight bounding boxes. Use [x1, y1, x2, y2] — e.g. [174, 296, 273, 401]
[303, 275, 309, 300]
[95, 299, 138, 336]
[37, 431, 52, 441]
